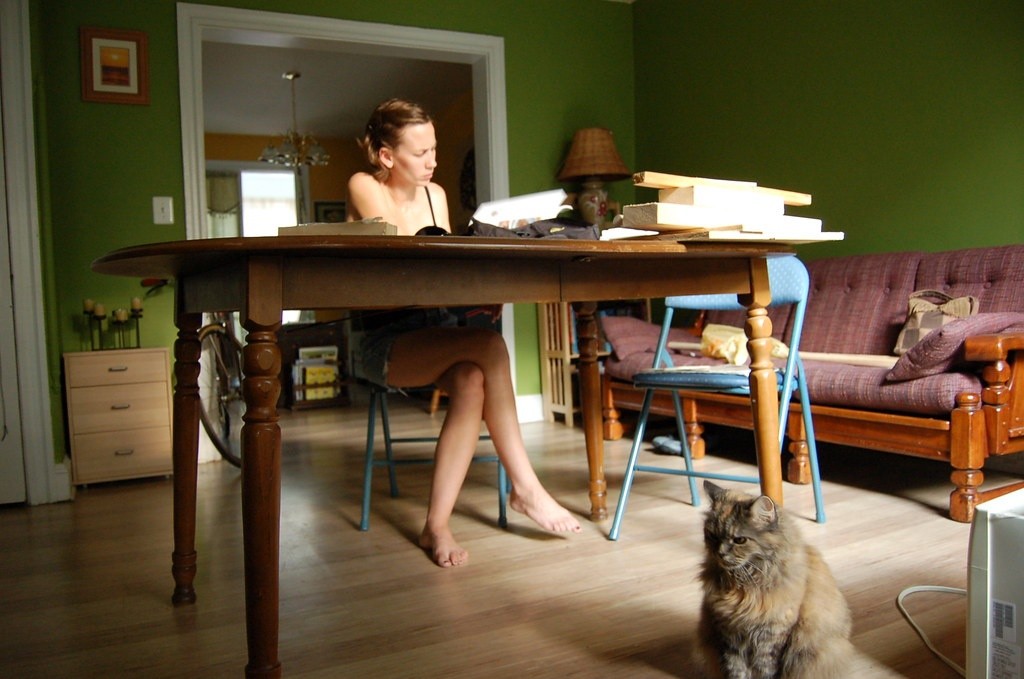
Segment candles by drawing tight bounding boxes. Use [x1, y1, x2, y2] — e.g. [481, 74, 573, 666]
[84, 299, 94, 311]
[95, 304, 104, 314]
[133, 297, 141, 309]
[116, 309, 128, 320]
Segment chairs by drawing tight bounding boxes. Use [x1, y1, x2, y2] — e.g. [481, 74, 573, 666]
[608, 253, 826, 541]
[361, 380, 512, 531]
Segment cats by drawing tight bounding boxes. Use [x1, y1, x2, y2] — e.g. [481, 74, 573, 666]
[690, 480, 908, 679]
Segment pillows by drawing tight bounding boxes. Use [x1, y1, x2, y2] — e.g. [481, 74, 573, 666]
[601, 316, 703, 361]
[885, 311, 1022, 381]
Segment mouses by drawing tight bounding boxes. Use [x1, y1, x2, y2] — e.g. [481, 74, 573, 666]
[415, 226, 448, 237]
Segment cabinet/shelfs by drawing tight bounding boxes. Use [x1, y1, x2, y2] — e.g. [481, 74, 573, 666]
[538, 190, 652, 429]
[63, 347, 174, 488]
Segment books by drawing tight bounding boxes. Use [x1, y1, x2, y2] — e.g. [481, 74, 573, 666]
[469, 189, 573, 231]
[277, 222, 398, 235]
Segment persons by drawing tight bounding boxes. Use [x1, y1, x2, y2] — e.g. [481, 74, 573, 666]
[348, 97, 582, 569]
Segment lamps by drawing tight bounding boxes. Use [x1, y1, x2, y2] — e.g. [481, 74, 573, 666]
[559, 127, 631, 226]
[258, 69, 329, 167]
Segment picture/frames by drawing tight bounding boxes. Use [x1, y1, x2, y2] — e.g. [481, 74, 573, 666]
[314, 201, 346, 222]
[80, 24, 150, 105]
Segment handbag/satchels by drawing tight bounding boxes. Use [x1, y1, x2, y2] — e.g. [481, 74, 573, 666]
[894, 288, 978, 355]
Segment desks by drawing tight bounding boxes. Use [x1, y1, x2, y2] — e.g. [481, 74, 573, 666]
[89, 234, 784, 678]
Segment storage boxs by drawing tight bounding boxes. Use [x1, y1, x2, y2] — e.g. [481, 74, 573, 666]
[278, 222, 397, 235]
[622, 185, 822, 233]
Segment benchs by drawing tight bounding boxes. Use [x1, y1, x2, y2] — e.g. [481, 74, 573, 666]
[602, 245, 1024, 522]
